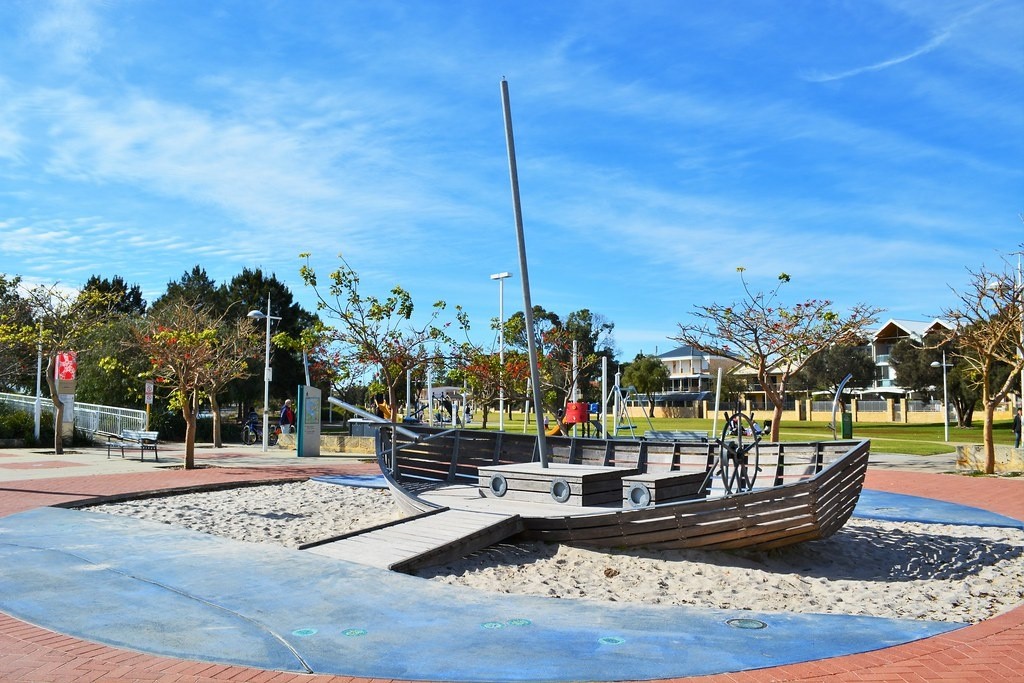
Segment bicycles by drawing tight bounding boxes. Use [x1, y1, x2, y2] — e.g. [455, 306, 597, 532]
[241, 419, 278, 446]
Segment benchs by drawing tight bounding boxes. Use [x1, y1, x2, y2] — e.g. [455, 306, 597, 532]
[104, 429, 161, 463]
[640, 430, 709, 443]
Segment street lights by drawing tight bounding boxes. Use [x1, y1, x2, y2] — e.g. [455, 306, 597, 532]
[247, 292, 282, 453]
[986, 252, 1024, 395]
[33, 291, 53, 442]
[930, 349, 955, 441]
[490, 272, 514, 433]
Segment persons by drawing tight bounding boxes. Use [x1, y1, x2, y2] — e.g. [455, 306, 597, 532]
[729, 418, 771, 436]
[279, 399, 295, 434]
[544, 416, 549, 430]
[398, 405, 471, 424]
[1012, 408, 1022, 448]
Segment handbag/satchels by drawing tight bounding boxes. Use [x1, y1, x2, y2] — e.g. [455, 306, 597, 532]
[278, 434, 294, 446]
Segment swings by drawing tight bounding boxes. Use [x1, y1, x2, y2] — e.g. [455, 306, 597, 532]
[617, 390, 638, 430]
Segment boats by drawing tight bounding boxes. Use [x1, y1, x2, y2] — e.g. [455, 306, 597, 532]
[375, 399, 872, 554]
